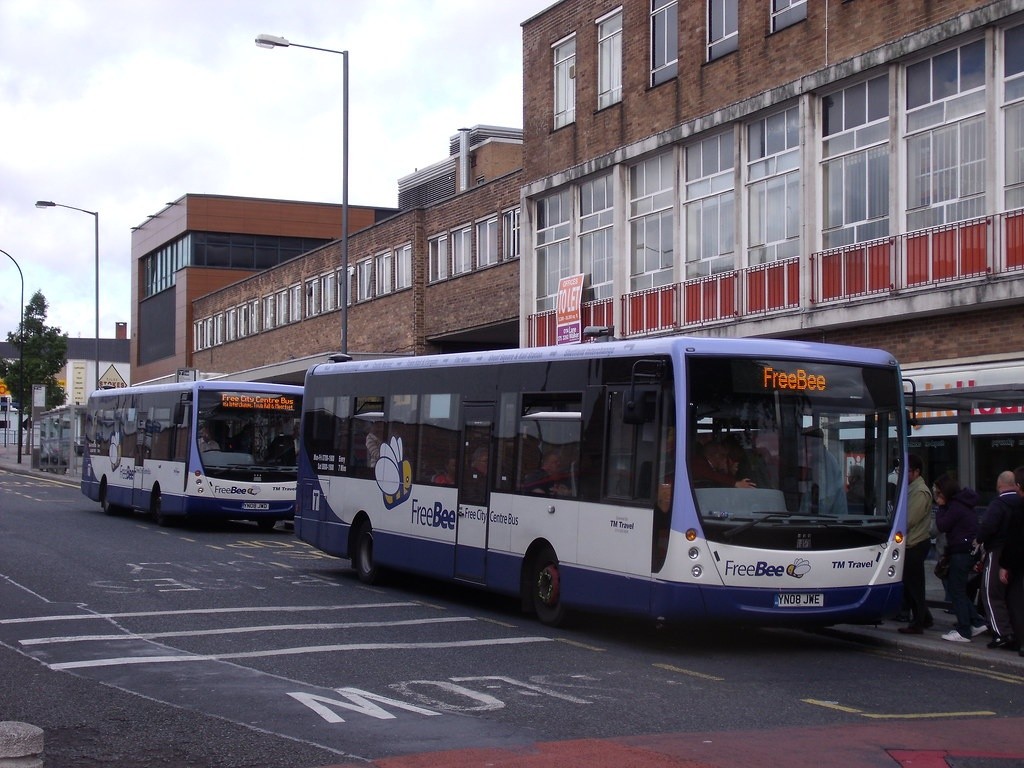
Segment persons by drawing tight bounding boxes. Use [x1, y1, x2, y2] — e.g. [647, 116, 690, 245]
[197, 409, 300, 466]
[364, 421, 1024, 658]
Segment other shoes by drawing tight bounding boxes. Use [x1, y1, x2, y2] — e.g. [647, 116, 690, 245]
[898, 624, 922, 635]
[923, 620, 935, 629]
[987, 635, 1013, 648]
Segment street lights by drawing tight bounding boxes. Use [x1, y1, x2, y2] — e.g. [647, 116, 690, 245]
[252, 33, 351, 356]
[34, 199, 102, 391]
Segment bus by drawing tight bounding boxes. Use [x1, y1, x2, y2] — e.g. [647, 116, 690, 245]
[78, 380, 325, 530]
[291, 336, 920, 629]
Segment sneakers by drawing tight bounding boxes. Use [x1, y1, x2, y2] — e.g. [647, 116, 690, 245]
[970, 625, 988, 637]
[941, 630, 971, 642]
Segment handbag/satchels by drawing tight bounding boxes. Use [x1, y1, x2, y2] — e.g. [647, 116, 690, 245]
[934, 556, 949, 579]
